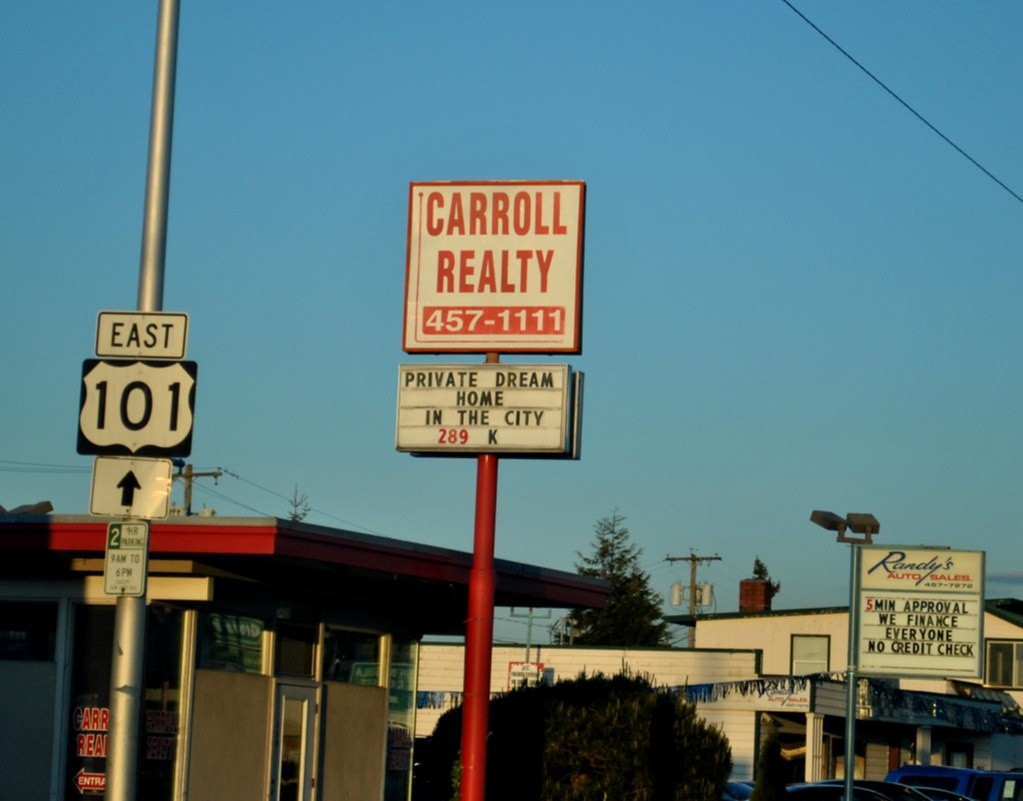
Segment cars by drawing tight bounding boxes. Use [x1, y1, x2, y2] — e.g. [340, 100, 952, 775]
[712, 763, 1022, 801]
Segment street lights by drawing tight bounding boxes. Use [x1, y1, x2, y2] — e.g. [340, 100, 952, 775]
[808, 508, 881, 801]
